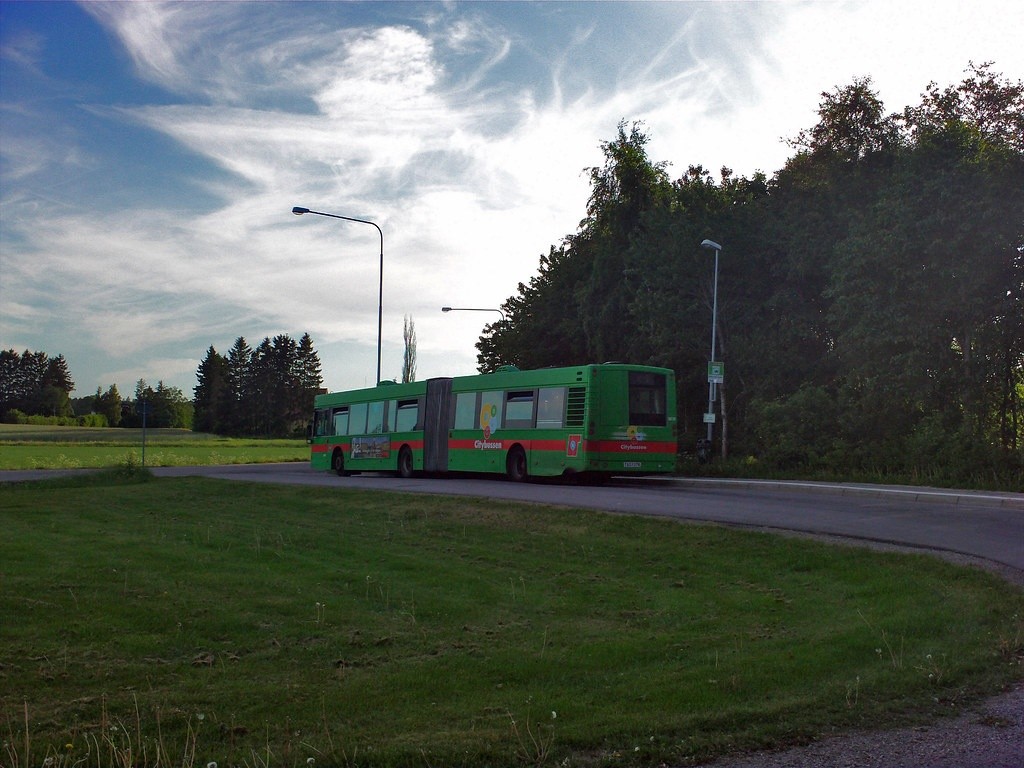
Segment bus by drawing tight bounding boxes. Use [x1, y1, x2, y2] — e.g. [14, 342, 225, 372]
[305, 361, 679, 484]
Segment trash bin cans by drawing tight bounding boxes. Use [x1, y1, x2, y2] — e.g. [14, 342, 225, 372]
[696, 439, 714, 465]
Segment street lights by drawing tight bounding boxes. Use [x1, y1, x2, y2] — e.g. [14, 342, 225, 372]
[291, 207, 382, 384]
[700, 239, 722, 439]
[442, 309, 505, 331]
